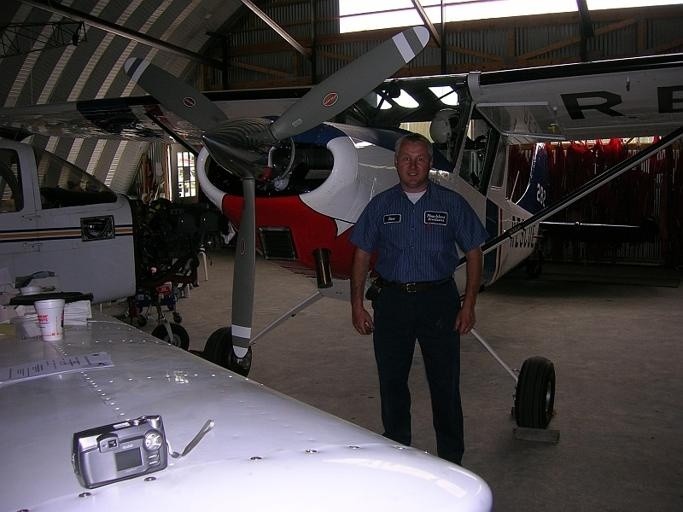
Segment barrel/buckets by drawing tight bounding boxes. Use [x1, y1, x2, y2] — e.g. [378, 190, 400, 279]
[35, 299, 65, 341]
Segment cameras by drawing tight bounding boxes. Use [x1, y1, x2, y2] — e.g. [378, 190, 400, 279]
[72, 415, 168, 489]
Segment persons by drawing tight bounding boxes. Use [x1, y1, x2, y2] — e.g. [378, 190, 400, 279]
[348, 132, 490, 466]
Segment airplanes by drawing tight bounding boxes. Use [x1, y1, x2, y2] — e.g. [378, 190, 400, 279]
[1, 26, 682, 431]
[1, 136, 239, 352]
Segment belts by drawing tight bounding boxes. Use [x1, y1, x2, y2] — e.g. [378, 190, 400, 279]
[372, 277, 455, 294]
